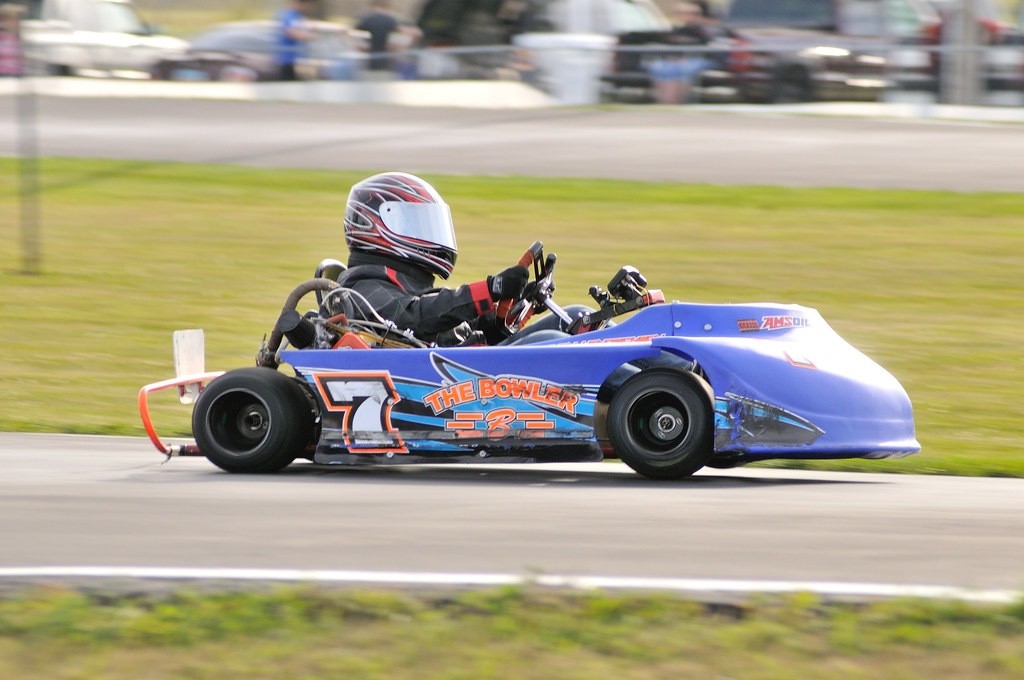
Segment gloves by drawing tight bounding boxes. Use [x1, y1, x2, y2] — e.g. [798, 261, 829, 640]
[522, 280, 556, 315]
[487, 266, 530, 301]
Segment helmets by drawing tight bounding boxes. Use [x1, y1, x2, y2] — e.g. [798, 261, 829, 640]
[343, 172, 458, 280]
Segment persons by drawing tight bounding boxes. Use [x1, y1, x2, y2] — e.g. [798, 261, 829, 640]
[0, 2, 29, 78]
[336, 171, 618, 346]
[637, 34, 717, 105]
[266, 0, 349, 81]
[353, 0, 424, 73]
